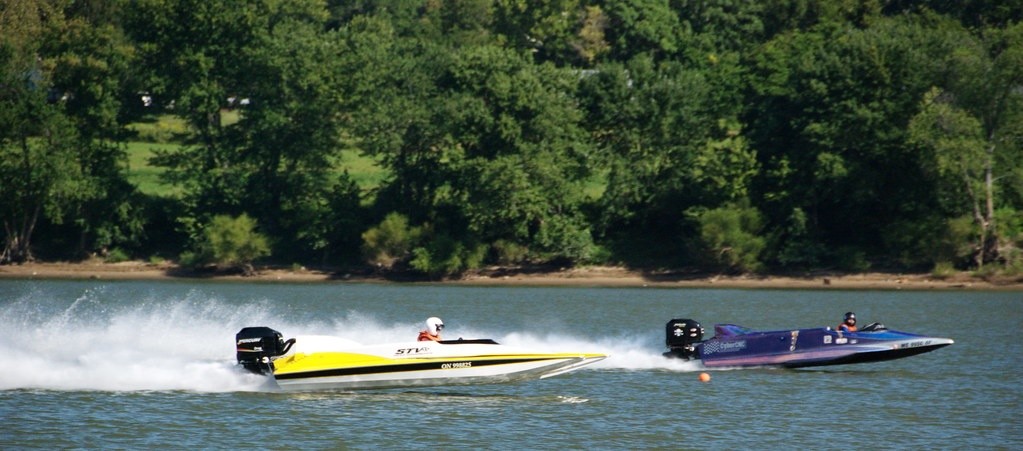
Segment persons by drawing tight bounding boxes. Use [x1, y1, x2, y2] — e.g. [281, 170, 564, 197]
[837, 312, 858, 332]
[417, 317, 445, 341]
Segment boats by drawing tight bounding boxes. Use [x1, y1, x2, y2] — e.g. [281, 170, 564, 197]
[662, 320, 955, 366]
[236, 327, 608, 389]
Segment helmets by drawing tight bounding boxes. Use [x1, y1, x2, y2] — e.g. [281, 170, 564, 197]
[425, 317, 445, 337]
[844, 312, 856, 326]
